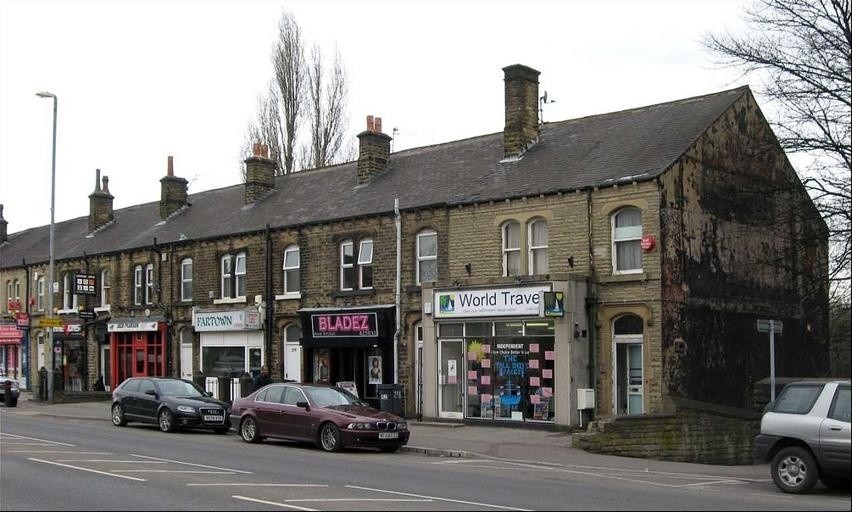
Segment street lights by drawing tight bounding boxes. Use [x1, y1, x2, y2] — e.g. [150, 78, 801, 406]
[34, 89, 63, 403]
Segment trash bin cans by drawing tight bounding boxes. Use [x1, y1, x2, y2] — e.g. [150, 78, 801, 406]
[377, 383, 405, 418]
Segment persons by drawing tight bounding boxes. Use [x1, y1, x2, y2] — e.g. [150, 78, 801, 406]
[253, 365, 272, 393]
[319, 357, 329, 379]
[371, 357, 380, 379]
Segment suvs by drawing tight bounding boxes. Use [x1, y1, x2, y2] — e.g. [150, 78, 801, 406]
[0, 366, 22, 409]
[747, 377, 852, 495]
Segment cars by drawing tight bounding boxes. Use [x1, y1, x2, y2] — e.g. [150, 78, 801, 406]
[229, 380, 413, 454]
[109, 374, 231, 436]
